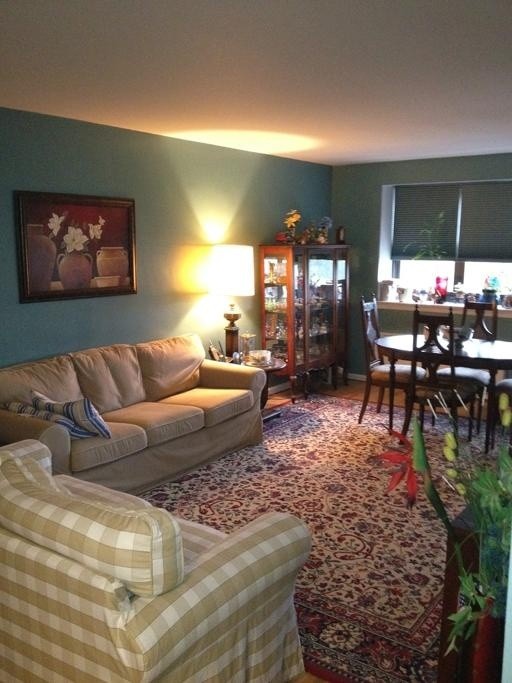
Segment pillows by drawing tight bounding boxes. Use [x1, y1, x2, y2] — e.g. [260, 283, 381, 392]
[4, 387, 112, 443]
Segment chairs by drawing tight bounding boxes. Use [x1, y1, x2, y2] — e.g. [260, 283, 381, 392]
[0, 437, 314, 682]
[358, 291, 499, 444]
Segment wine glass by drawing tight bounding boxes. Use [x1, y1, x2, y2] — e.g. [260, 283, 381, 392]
[442, 326, 475, 350]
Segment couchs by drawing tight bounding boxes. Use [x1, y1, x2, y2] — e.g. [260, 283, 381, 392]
[1, 333, 268, 498]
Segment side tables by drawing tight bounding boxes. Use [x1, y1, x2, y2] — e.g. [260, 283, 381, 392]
[222, 353, 288, 422]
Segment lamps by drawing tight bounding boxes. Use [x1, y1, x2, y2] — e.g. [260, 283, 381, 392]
[206, 241, 256, 357]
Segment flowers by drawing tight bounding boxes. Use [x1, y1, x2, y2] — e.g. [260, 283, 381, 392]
[275, 206, 302, 242]
[46, 210, 107, 255]
[366, 388, 510, 659]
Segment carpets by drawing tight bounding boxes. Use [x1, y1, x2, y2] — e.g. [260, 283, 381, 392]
[138, 391, 510, 682]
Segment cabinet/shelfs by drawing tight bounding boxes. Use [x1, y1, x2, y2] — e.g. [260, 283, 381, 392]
[258, 241, 352, 405]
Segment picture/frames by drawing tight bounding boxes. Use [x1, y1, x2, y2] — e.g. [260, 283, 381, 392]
[12, 188, 139, 304]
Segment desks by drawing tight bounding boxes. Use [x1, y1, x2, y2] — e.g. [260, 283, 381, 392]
[373, 333, 512, 454]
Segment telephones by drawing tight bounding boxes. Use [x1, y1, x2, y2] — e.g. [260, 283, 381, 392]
[208, 339, 220, 361]
